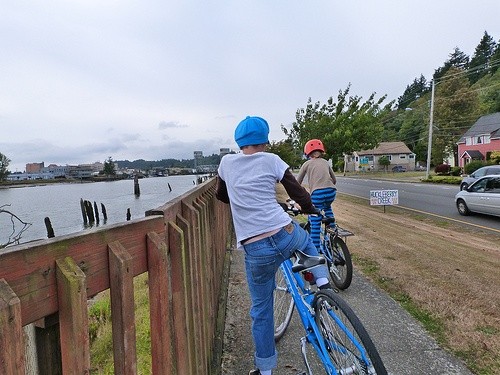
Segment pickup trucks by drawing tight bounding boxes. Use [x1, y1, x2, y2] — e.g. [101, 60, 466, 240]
[392, 165, 406, 173]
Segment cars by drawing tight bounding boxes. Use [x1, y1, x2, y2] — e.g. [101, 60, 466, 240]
[454, 174, 500, 217]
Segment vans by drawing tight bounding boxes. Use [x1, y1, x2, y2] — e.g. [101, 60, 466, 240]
[459, 164, 500, 192]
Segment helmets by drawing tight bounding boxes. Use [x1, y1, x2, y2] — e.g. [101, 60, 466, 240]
[303, 139, 325, 158]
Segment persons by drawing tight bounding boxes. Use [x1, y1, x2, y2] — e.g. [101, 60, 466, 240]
[215, 115, 340, 375]
[287, 140, 337, 255]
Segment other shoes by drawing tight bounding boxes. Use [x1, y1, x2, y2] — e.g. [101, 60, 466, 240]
[249, 368, 262, 375]
[321, 288, 338, 307]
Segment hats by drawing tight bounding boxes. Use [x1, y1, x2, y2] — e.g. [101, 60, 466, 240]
[234, 115, 270, 149]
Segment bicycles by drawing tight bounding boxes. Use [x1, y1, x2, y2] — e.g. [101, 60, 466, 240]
[285, 196, 357, 291]
[261, 197, 391, 375]
